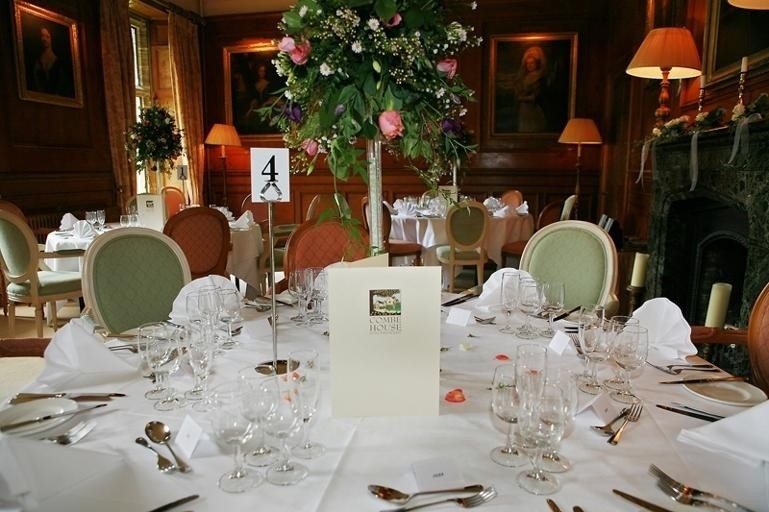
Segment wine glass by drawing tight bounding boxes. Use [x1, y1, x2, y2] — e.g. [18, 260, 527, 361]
[137, 310, 212, 412]
[490, 346, 577, 495]
[577, 301, 650, 407]
[289, 263, 329, 327]
[499, 270, 565, 341]
[206, 350, 326, 495]
[187, 283, 240, 352]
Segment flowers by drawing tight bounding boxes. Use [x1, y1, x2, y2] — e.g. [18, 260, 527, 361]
[276, 2, 485, 185]
[120, 105, 189, 172]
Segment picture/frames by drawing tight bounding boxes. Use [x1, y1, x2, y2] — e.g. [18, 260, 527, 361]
[490, 31, 580, 144]
[221, 35, 298, 144]
[12, 0, 88, 110]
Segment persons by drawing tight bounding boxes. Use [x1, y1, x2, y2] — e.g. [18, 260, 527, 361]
[247, 65, 285, 130]
[32, 27, 61, 93]
[508, 44, 559, 132]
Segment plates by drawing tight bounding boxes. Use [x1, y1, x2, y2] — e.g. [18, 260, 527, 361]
[680, 372, 766, 408]
[1, 399, 79, 439]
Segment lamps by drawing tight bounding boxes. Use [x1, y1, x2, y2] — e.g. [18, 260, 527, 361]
[204, 122, 243, 211]
[553, 118, 604, 222]
[625, 27, 703, 141]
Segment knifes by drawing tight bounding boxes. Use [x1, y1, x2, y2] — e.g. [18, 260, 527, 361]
[657, 402, 724, 425]
[133, 494, 201, 511]
[0, 404, 115, 431]
[610, 484, 673, 512]
[657, 375, 752, 386]
[548, 305, 584, 325]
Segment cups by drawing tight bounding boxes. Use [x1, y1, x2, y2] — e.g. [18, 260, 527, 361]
[82, 208, 144, 229]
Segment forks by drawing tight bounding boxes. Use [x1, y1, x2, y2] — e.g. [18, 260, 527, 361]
[605, 404, 643, 445]
[135, 435, 178, 475]
[645, 463, 760, 512]
[382, 486, 498, 511]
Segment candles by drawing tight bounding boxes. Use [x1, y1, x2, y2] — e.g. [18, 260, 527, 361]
[741, 57, 750, 73]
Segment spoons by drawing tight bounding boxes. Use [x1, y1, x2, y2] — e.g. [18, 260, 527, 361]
[591, 405, 628, 436]
[146, 419, 190, 475]
[365, 481, 485, 504]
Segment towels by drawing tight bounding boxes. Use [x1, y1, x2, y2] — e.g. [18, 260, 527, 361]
[674, 400, 769, 469]
[0, 431, 124, 511]
